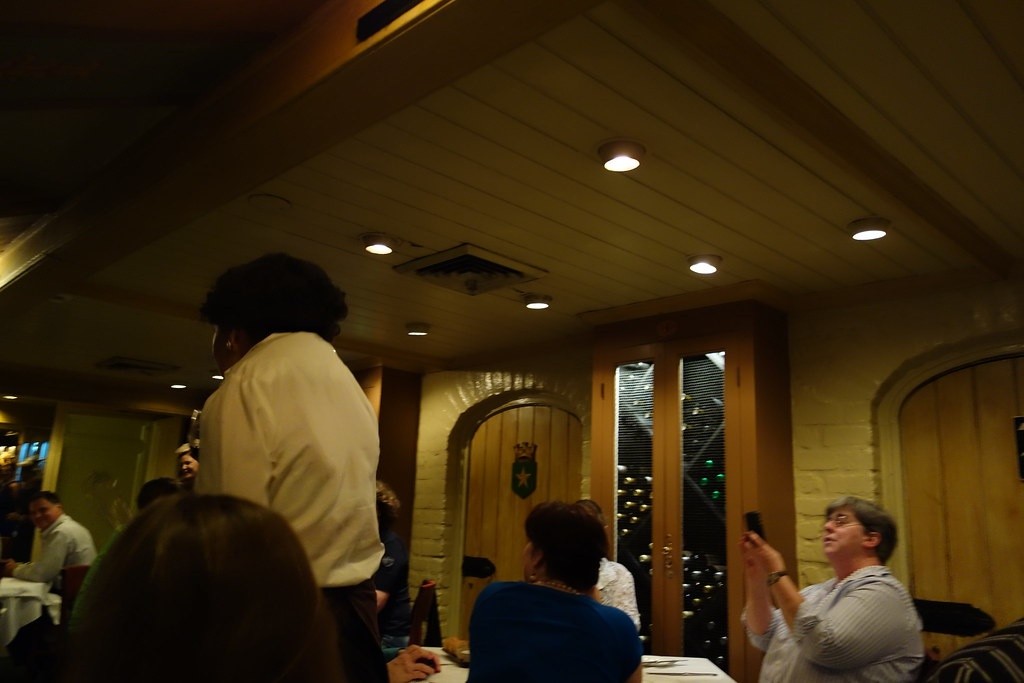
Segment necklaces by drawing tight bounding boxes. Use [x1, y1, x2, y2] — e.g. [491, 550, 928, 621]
[545, 581, 577, 592]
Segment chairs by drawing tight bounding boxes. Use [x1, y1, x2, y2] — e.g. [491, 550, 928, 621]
[411, 580, 442, 647]
[61, 566, 89, 633]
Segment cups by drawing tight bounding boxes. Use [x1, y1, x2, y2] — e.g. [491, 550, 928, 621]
[188, 410, 201, 457]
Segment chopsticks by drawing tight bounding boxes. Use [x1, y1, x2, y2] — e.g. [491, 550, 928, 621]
[641, 659, 690, 667]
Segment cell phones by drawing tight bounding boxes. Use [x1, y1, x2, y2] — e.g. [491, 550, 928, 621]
[745, 511, 764, 540]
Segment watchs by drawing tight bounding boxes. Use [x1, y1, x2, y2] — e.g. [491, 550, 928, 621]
[767, 571, 787, 586]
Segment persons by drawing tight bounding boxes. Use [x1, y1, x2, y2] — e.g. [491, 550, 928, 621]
[64, 495, 439, 683]
[0, 456, 37, 562]
[373, 482, 410, 637]
[67, 478, 184, 633]
[4, 491, 97, 593]
[741, 496, 924, 683]
[466, 501, 642, 683]
[176, 443, 200, 490]
[193, 253, 385, 646]
[576, 499, 641, 630]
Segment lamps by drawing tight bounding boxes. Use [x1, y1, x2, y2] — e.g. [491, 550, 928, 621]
[406, 322, 429, 336]
[522, 293, 553, 309]
[686, 250, 724, 275]
[845, 214, 892, 241]
[596, 135, 650, 172]
[361, 232, 403, 255]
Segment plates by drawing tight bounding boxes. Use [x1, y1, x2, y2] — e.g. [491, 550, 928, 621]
[442, 647, 470, 666]
[641, 655, 718, 676]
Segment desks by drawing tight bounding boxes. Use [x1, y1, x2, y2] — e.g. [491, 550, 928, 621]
[0, 577, 63, 658]
[410, 647, 737, 683]
